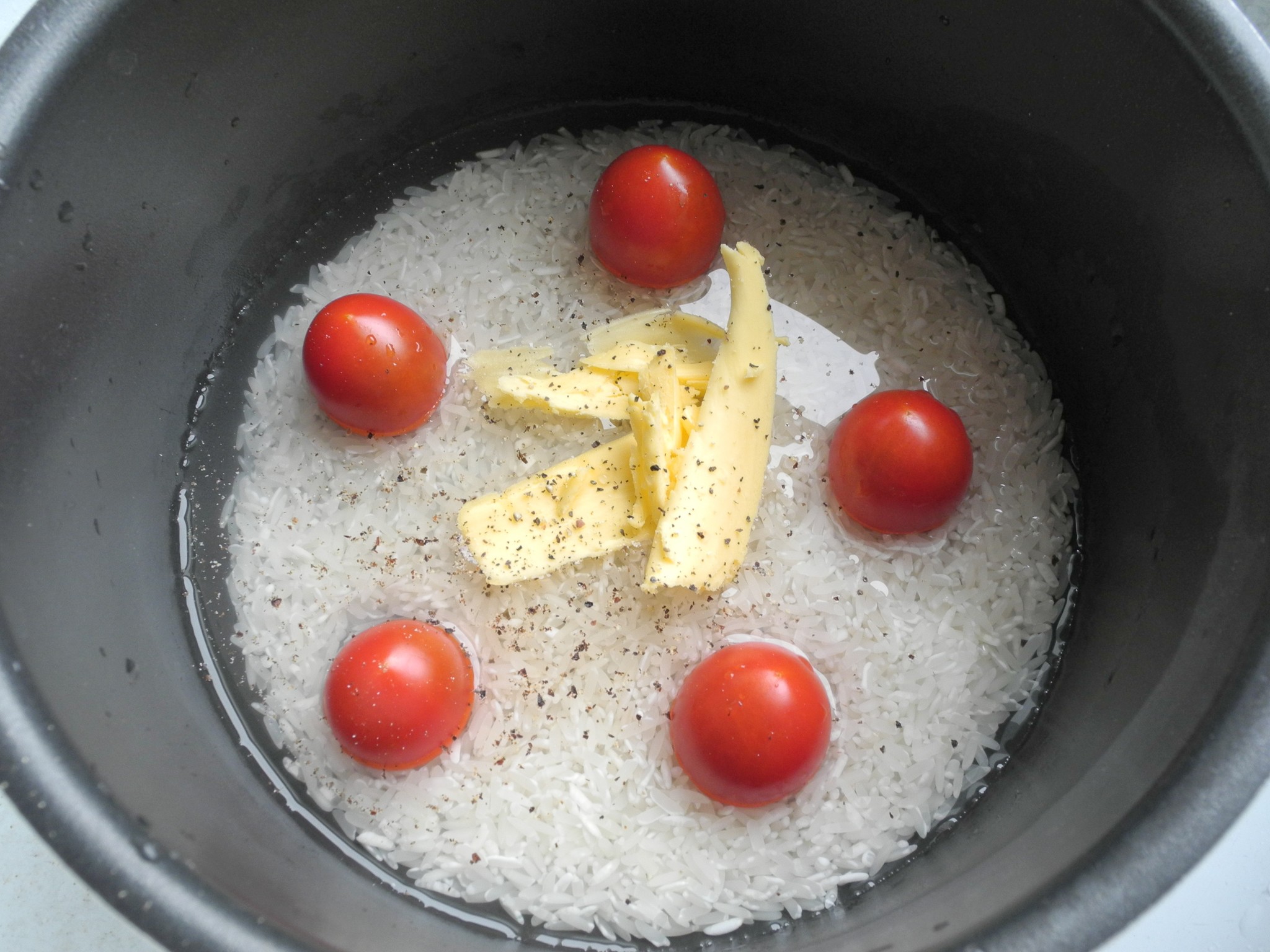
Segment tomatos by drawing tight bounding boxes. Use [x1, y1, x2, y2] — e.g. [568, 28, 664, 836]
[668, 640, 831, 806]
[588, 144, 726, 287]
[323, 619, 474, 769]
[302, 293, 447, 436]
[828, 389, 974, 536]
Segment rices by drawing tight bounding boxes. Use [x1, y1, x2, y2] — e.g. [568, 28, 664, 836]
[213, 120, 1077, 947]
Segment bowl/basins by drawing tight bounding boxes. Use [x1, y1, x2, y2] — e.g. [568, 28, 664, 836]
[0, 1, 1270, 952]
